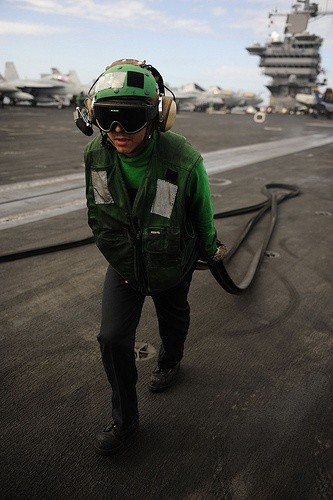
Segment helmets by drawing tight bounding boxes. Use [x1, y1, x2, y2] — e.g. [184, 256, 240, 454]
[91, 63, 160, 102]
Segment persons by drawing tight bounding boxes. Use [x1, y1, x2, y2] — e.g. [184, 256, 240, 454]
[84, 59, 228, 458]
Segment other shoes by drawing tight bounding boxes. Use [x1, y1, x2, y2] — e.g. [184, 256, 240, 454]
[148, 359, 182, 391]
[93, 410, 139, 456]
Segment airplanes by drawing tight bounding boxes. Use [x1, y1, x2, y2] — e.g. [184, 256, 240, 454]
[0, 60, 333, 122]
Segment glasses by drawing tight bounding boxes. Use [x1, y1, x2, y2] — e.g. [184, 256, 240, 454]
[93, 102, 158, 134]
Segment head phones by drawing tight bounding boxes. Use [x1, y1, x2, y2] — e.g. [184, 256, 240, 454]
[72, 60, 178, 136]
